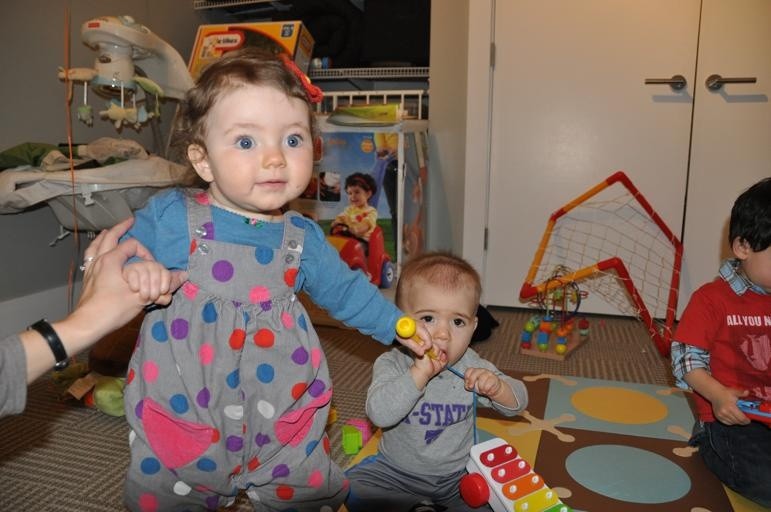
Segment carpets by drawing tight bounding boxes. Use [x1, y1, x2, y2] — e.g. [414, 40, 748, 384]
[337, 368, 771, 512]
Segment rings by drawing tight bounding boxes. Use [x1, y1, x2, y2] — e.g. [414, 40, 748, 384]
[78, 250, 95, 269]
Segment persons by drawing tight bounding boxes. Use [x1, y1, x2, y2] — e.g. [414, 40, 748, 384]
[105, 49, 430, 508]
[332, 172, 376, 242]
[2, 238, 191, 421]
[680, 173, 771, 504]
[346, 250, 532, 509]
[374, 129, 400, 237]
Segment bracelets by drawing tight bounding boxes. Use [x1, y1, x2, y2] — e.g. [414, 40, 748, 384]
[28, 315, 71, 374]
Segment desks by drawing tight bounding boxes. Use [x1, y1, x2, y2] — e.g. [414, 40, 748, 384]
[288, 113, 433, 332]
